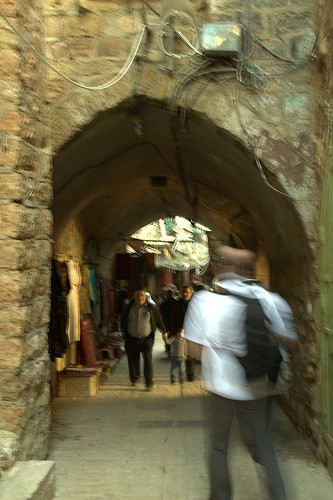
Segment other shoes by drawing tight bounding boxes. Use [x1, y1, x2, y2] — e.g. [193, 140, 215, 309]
[145, 386, 155, 391]
[187, 373, 196, 381]
[131, 381, 140, 386]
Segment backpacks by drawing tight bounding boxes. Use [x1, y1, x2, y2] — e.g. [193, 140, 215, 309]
[199, 286, 292, 395]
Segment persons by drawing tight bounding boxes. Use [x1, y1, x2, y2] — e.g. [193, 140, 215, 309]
[165, 329, 186, 386]
[143, 278, 211, 356]
[120, 288, 168, 391]
[184, 244, 296, 500]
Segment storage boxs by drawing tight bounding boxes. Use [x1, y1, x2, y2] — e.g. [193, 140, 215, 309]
[89, 358, 119, 381]
[55, 365, 104, 398]
